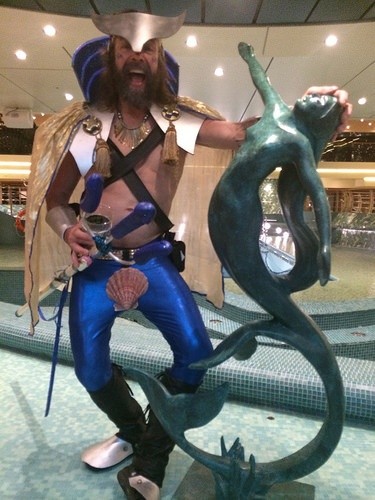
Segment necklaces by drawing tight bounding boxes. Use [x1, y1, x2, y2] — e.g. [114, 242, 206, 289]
[112, 101, 152, 150]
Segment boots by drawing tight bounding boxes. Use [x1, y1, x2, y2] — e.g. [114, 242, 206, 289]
[115, 379, 203, 500]
[78, 363, 147, 473]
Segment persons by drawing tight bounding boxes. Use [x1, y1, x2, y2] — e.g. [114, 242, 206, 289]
[43, 8, 353, 500]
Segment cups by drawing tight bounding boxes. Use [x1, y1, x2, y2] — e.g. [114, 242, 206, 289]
[79, 206, 113, 239]
